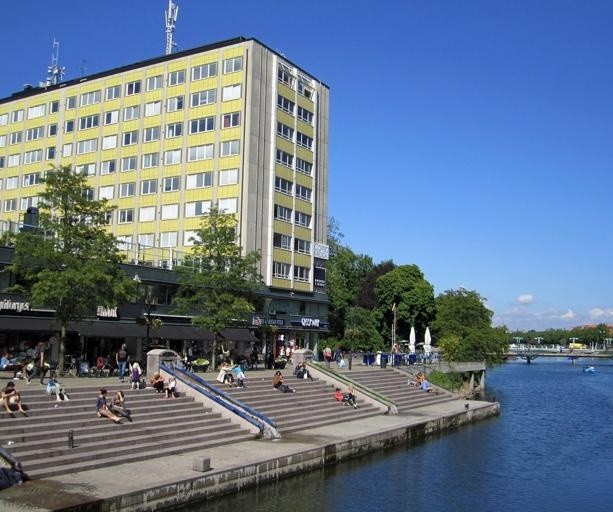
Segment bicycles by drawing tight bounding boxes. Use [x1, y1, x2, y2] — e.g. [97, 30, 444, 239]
[63, 353, 78, 376]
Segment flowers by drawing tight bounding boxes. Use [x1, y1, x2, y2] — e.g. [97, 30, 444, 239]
[189, 357, 209, 365]
[272, 356, 286, 363]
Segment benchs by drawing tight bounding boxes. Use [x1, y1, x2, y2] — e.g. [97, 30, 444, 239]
[0, 368, 19, 379]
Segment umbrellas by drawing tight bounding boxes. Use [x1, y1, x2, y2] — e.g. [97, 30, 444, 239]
[408, 325, 415, 353]
[423, 327, 431, 355]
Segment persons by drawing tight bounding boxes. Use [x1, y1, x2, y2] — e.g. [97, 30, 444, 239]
[178, 347, 312, 394]
[335, 387, 350, 405]
[46, 373, 69, 401]
[91, 342, 176, 424]
[333, 345, 342, 366]
[0, 341, 54, 386]
[585, 362, 593, 368]
[392, 343, 399, 354]
[0, 382, 25, 414]
[323, 345, 332, 368]
[348, 393, 359, 408]
[408, 370, 431, 392]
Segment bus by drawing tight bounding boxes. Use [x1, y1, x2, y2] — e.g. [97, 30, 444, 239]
[569, 342, 586, 349]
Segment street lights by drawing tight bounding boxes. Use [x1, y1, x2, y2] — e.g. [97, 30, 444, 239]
[569, 337, 579, 349]
[512, 337, 524, 349]
[144, 289, 156, 371]
[391, 303, 397, 356]
[534, 337, 544, 348]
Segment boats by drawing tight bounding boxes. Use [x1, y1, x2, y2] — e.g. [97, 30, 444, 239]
[583, 366, 595, 372]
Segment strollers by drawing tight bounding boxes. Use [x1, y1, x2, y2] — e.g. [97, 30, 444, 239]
[89, 356, 109, 378]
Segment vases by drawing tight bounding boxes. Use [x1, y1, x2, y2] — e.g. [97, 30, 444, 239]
[273, 361, 285, 369]
[192, 364, 208, 373]
